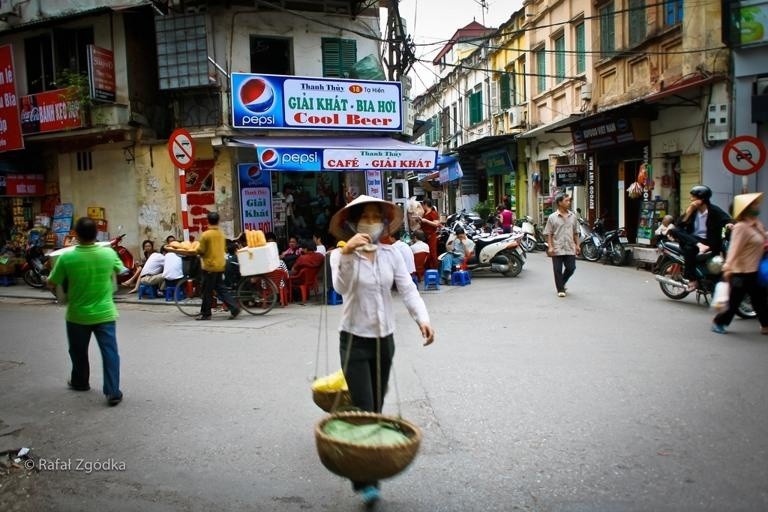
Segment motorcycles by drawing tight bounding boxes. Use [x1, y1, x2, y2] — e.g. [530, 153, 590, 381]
[653, 226, 758, 319]
[436, 208, 548, 278]
[109, 232, 134, 285]
[23, 237, 50, 288]
[575, 207, 600, 262]
[591, 214, 629, 266]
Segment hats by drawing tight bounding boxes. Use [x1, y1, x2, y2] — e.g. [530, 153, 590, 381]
[330, 194, 405, 243]
[733, 192, 765, 221]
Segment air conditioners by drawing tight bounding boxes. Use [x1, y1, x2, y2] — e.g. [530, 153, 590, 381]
[508, 105, 527, 129]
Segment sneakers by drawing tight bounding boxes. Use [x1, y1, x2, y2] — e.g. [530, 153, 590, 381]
[107, 391, 122, 407]
[712, 324, 727, 335]
[760, 326, 768, 334]
[558, 291, 566, 297]
[362, 486, 380, 504]
[195, 314, 212, 320]
[67, 379, 91, 393]
[227, 309, 241, 320]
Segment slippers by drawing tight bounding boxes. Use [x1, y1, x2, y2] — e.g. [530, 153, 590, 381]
[698, 251, 713, 256]
[687, 280, 696, 292]
[121, 281, 131, 288]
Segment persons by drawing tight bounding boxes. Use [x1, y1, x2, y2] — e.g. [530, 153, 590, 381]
[543, 192, 581, 297]
[328, 192, 436, 510]
[120, 235, 176, 295]
[195, 211, 240, 321]
[498, 195, 517, 233]
[221, 225, 326, 309]
[46, 216, 123, 407]
[655, 183, 766, 335]
[389, 199, 477, 291]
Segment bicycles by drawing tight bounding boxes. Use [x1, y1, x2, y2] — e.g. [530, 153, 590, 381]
[174, 254, 278, 317]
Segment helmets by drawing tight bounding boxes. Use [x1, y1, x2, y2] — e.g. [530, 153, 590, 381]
[689, 185, 712, 202]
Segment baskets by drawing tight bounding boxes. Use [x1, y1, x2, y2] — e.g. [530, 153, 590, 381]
[315, 411, 422, 481]
[312, 374, 358, 416]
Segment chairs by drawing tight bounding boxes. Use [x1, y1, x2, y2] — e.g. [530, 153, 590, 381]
[425, 233, 440, 268]
[410, 252, 430, 283]
[455, 251, 474, 271]
[184, 265, 325, 312]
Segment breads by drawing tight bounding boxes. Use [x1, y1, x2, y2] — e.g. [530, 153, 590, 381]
[166, 240, 199, 251]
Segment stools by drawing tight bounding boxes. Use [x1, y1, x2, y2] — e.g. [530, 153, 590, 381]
[326, 287, 343, 305]
[410, 274, 419, 288]
[424, 269, 440, 290]
[166, 285, 186, 302]
[452, 270, 471, 285]
[138, 285, 157, 299]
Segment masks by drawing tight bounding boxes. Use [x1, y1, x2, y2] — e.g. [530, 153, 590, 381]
[355, 223, 384, 243]
